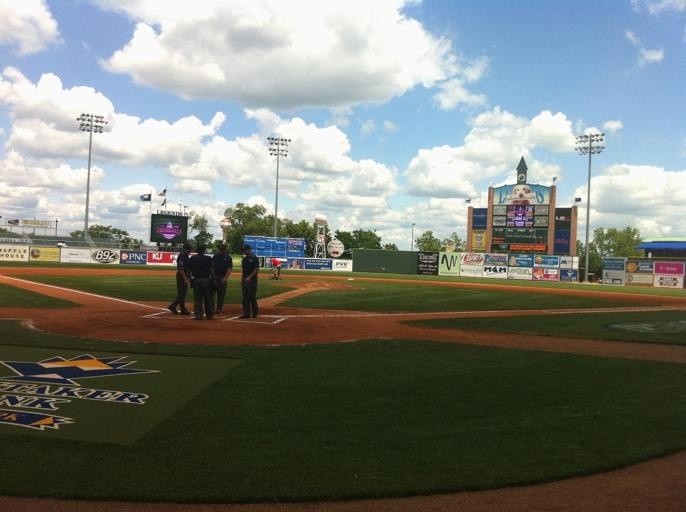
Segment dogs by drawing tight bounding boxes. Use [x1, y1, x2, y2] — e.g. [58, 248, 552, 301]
[510, 184, 531, 206]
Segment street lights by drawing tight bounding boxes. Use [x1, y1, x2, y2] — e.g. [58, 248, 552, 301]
[76, 113, 109, 238]
[411, 222, 416, 251]
[267, 136, 292, 237]
[574, 132, 606, 283]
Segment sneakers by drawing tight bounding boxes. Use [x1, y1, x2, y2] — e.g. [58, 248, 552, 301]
[252, 306, 258, 318]
[181, 310, 191, 314]
[194, 308, 224, 320]
[239, 313, 250, 318]
[168, 305, 178, 313]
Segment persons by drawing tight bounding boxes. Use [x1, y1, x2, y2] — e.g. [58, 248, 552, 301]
[188, 244, 214, 320]
[270, 256, 281, 280]
[240, 245, 258, 318]
[212, 244, 232, 314]
[167, 243, 194, 315]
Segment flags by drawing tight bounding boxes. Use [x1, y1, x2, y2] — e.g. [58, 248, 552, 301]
[159, 199, 166, 206]
[158, 188, 167, 196]
[139, 194, 151, 202]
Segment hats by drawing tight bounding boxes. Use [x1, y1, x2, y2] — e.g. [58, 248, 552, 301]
[194, 245, 206, 250]
[216, 244, 226, 249]
[183, 244, 194, 249]
[240, 244, 252, 251]
